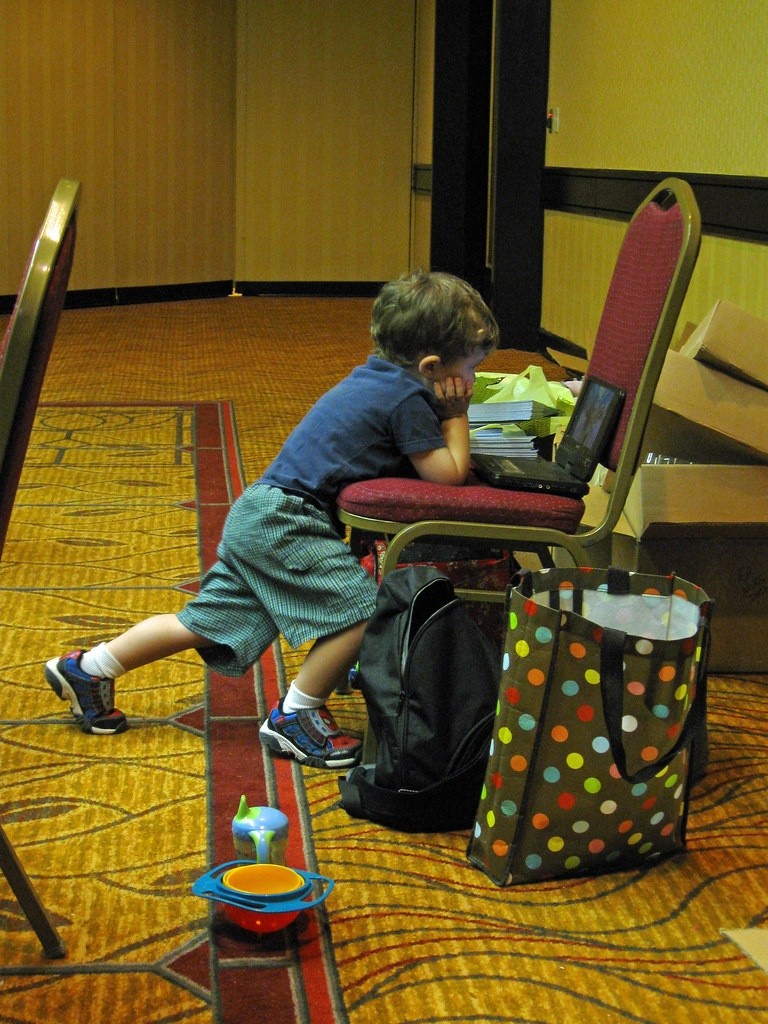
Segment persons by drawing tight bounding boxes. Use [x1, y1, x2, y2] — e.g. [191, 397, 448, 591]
[43, 272, 502, 768]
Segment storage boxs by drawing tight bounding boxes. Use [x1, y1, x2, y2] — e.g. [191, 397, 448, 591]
[544, 299, 768, 676]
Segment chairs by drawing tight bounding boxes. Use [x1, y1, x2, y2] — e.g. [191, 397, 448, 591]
[335, 176, 704, 767]
[0, 172, 83, 959]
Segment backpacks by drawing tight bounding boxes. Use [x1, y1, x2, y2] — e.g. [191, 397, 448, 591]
[335, 565, 509, 835]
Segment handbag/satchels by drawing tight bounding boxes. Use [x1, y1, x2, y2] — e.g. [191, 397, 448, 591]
[464, 565, 709, 887]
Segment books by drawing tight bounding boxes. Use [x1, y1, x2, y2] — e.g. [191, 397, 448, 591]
[467, 400, 559, 422]
[468, 429, 539, 461]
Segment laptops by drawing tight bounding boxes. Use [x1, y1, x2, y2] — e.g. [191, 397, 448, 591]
[470, 374, 626, 496]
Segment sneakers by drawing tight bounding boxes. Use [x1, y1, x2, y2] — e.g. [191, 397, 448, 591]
[43, 648, 128, 735]
[257, 697, 366, 768]
